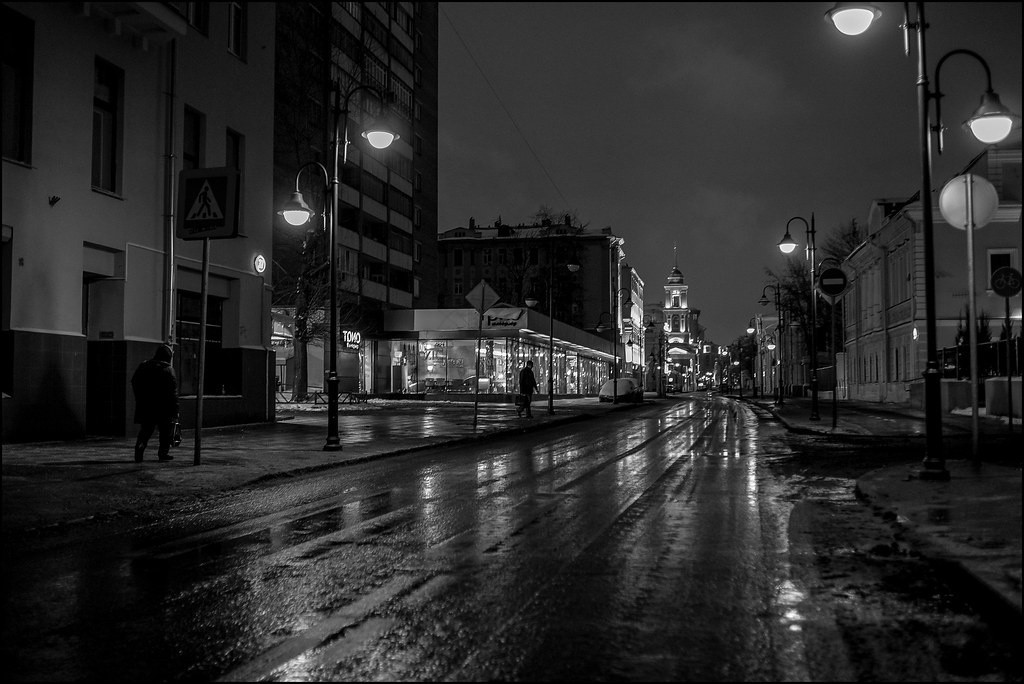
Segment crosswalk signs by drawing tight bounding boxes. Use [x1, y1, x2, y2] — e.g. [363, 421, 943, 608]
[174, 163, 244, 243]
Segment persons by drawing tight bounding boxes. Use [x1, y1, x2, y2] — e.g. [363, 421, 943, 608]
[133, 344, 179, 463]
[516, 360, 538, 419]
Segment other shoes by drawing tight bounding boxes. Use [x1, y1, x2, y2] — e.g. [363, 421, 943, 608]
[134, 444, 143, 461]
[516, 407, 522, 418]
[158, 455, 175, 462]
[526, 414, 534, 419]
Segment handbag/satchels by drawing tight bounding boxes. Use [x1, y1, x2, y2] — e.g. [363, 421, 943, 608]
[515, 393, 531, 407]
[169, 413, 183, 448]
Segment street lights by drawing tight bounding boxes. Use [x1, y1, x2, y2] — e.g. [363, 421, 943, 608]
[594, 277, 635, 404]
[746, 316, 776, 402]
[524, 249, 583, 412]
[707, 339, 751, 398]
[276, 75, 402, 451]
[776, 211, 850, 419]
[758, 282, 802, 407]
[823, 1, 1014, 478]
[627, 313, 693, 400]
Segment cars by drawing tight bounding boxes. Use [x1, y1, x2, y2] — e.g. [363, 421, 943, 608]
[666, 385, 675, 394]
[599, 377, 644, 403]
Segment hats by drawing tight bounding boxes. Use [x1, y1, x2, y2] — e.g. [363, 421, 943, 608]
[155, 344, 174, 360]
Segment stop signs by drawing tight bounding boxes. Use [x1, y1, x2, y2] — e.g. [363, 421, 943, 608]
[819, 268, 848, 296]
